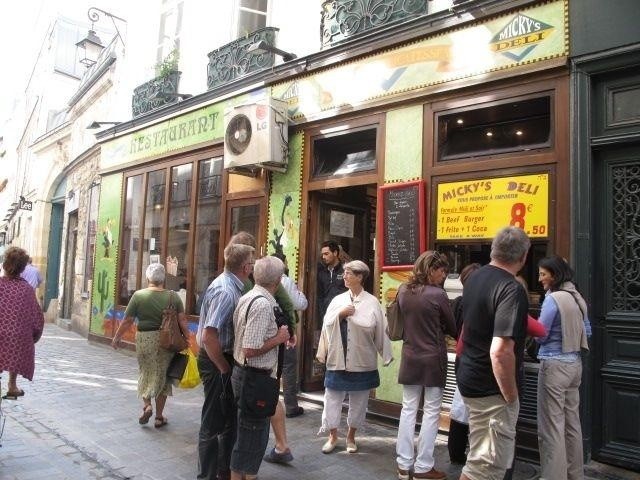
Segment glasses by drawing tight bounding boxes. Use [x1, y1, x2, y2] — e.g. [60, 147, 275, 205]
[244, 261, 255, 268]
[428, 248, 439, 268]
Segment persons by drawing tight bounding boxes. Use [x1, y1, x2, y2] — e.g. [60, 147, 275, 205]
[455, 277, 549, 479]
[271, 253, 308, 418]
[196, 244, 256, 480]
[1, 247, 44, 397]
[318, 240, 348, 328]
[175, 279, 199, 314]
[20, 257, 42, 290]
[448, 263, 481, 465]
[454, 226, 531, 480]
[112, 263, 190, 428]
[230, 255, 290, 480]
[534, 255, 592, 480]
[316, 259, 394, 453]
[224, 231, 297, 464]
[386, 250, 451, 480]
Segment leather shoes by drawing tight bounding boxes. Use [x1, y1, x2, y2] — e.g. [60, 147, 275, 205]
[413, 470, 446, 480]
[344, 439, 358, 455]
[321, 434, 339, 454]
[395, 465, 409, 478]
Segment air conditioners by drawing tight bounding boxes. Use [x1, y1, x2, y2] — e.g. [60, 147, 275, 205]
[223, 96, 291, 180]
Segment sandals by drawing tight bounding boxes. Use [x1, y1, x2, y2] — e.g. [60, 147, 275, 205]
[138, 404, 153, 425]
[154, 415, 169, 428]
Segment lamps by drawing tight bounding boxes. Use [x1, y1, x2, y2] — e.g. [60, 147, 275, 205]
[84, 120, 122, 131]
[248, 39, 300, 61]
[151, 88, 196, 100]
[73, 4, 128, 71]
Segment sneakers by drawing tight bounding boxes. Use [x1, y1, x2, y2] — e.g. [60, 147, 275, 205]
[285, 406, 304, 418]
[262, 443, 295, 463]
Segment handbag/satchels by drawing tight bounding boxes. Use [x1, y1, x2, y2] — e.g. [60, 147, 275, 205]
[383, 283, 405, 342]
[156, 286, 191, 354]
